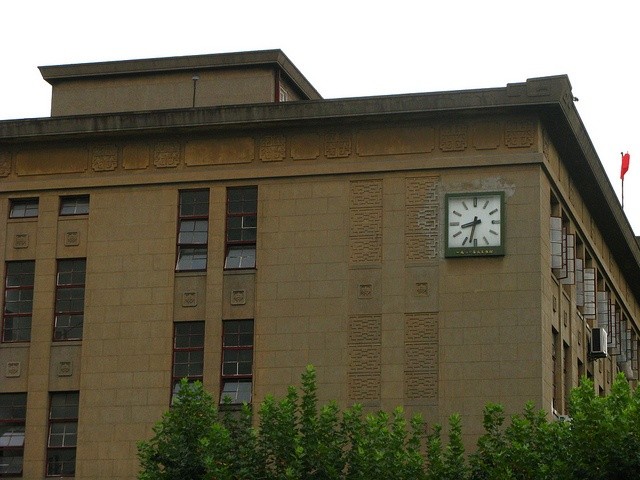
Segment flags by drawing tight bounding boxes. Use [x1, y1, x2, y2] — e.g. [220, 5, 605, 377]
[620, 153, 630, 178]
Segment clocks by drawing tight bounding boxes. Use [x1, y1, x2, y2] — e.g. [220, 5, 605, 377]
[444, 191, 505, 257]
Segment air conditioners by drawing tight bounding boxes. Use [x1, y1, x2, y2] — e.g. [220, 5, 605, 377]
[589, 327, 609, 354]
